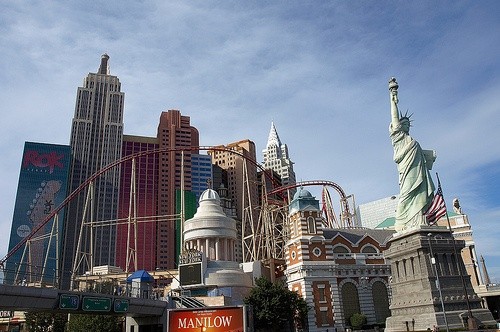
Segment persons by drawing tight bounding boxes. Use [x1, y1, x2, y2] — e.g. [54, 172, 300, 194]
[486, 283, 489, 292]
[430, 324, 440, 332]
[325, 324, 355, 332]
[387, 76, 439, 236]
[480, 296, 486, 309]
[14, 275, 166, 303]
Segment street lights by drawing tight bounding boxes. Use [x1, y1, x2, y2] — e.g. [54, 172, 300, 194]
[155, 268, 183, 309]
[427, 232, 449, 332]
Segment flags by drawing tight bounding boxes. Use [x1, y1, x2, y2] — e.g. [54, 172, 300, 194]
[420, 177, 447, 227]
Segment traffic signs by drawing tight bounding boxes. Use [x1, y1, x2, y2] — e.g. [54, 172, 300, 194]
[113, 299, 129, 312]
[82, 297, 111, 312]
[59, 294, 80, 309]
